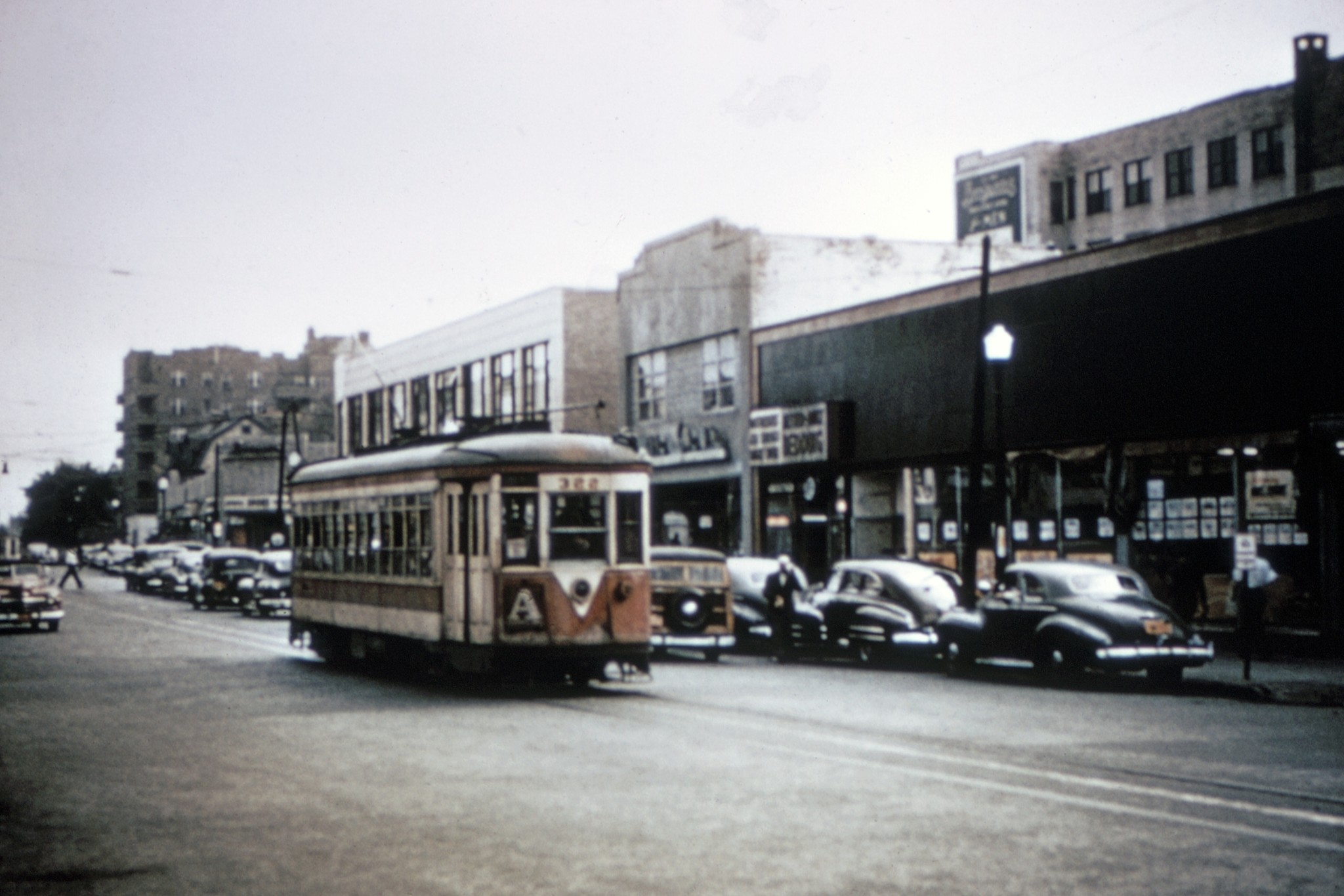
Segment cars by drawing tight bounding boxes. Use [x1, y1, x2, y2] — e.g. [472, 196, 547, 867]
[650, 545, 736, 663]
[0, 561, 65, 630]
[789, 558, 989, 670]
[726, 555, 835, 661]
[976, 559, 1212, 685]
[19, 542, 292, 617]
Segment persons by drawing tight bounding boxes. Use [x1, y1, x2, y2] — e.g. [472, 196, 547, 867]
[762, 551, 804, 665]
[1163, 545, 1212, 633]
[58, 544, 87, 592]
[554, 498, 739, 563]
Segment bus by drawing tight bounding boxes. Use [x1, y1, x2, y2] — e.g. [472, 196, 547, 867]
[287, 420, 652, 687]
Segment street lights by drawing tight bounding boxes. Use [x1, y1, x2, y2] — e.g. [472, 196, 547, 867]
[1218, 447, 1260, 681]
